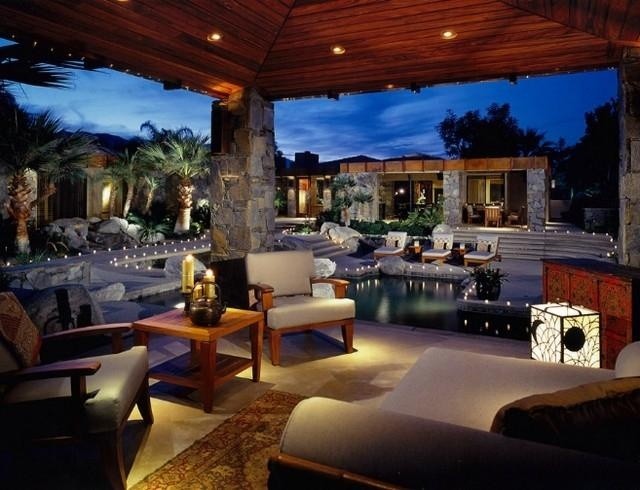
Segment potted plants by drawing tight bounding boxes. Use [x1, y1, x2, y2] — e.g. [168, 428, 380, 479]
[468, 262, 511, 301]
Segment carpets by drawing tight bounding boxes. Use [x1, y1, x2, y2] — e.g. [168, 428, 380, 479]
[127, 390, 320, 490]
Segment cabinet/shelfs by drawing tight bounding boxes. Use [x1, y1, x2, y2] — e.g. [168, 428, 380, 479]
[542, 261, 640, 369]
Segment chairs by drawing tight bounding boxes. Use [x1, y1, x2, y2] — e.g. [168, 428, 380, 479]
[463, 202, 527, 228]
[267, 341, 639, 489]
[245, 251, 356, 366]
[0, 292, 155, 490]
[373, 232, 502, 267]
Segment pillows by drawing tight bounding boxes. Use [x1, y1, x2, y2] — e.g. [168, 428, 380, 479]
[491, 374, 638, 462]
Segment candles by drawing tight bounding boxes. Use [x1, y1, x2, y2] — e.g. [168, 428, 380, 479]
[181, 253, 215, 298]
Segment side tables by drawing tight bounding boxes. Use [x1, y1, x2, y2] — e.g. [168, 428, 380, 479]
[133, 306, 265, 414]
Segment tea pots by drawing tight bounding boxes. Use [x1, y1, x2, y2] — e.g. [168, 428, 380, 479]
[184, 280, 228, 326]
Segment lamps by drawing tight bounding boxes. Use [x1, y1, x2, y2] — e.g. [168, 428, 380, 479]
[529, 297, 601, 369]
